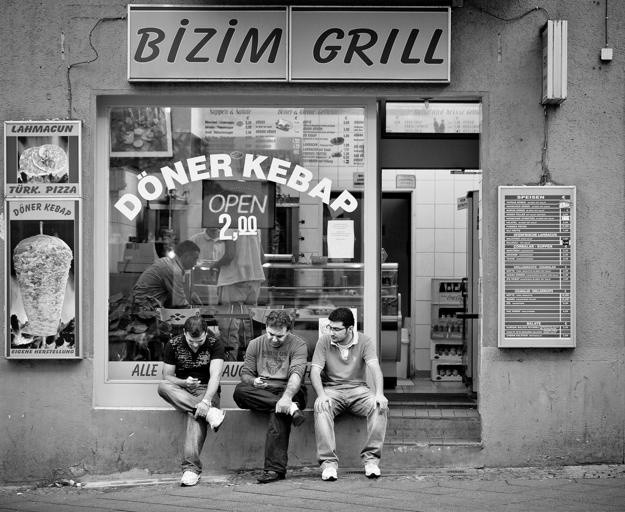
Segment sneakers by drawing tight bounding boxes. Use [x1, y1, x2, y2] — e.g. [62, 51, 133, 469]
[322, 468, 337, 481]
[180, 471, 201, 486]
[205, 407, 226, 433]
[364, 464, 381, 478]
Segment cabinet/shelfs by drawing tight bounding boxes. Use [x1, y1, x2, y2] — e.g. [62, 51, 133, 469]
[193, 262, 403, 362]
[431, 278, 466, 383]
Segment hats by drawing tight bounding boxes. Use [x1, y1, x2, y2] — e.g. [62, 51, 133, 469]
[19, 143, 69, 179]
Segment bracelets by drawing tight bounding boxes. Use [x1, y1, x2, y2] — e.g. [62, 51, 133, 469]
[202, 399, 211, 406]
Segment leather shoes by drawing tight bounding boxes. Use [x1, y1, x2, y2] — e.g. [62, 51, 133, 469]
[292, 409, 306, 427]
[257, 472, 285, 483]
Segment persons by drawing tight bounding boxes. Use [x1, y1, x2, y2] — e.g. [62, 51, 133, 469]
[233, 310, 308, 482]
[309, 307, 389, 481]
[157, 316, 227, 487]
[130, 240, 200, 324]
[188, 228, 266, 361]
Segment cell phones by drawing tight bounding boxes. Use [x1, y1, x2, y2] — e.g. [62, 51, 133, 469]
[260, 376, 270, 383]
[192, 378, 198, 382]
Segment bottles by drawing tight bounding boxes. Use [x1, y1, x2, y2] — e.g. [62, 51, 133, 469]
[438, 314, 464, 332]
[438, 347, 462, 357]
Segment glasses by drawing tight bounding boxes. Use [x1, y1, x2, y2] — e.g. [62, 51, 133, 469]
[326, 324, 346, 331]
